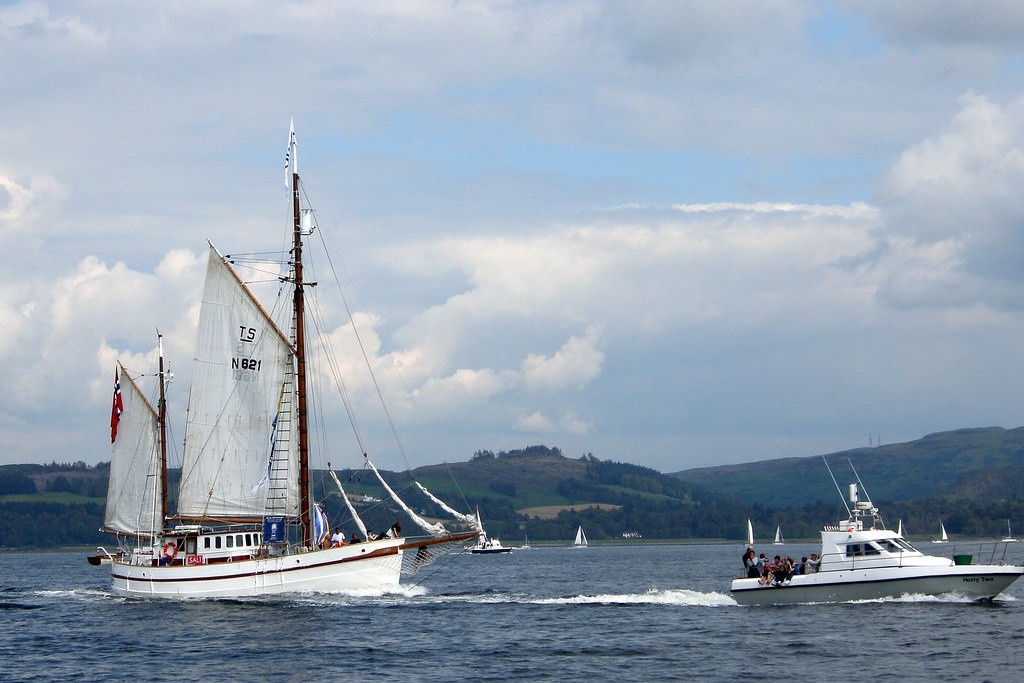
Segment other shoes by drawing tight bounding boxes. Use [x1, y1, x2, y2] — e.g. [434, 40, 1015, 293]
[758, 580, 763, 586]
[784, 580, 790, 585]
[776, 581, 781, 587]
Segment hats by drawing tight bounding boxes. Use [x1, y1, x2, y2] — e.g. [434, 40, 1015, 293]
[367, 529, 371, 535]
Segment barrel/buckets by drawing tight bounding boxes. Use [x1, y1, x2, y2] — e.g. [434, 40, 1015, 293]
[953, 555, 973, 565]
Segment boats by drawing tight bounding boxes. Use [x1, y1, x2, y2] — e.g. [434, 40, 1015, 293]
[571, 524, 590, 550]
[467, 536, 514, 556]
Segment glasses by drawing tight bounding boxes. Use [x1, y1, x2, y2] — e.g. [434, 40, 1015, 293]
[764, 561, 769, 562]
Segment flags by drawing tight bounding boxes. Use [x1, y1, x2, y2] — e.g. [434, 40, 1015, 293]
[111, 366, 123, 444]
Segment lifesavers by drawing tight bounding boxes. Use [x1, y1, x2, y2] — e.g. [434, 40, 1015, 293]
[331, 542, 346, 547]
[164, 542, 178, 558]
[482, 542, 485, 546]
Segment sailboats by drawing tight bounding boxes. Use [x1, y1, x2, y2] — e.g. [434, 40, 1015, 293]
[932, 519, 951, 545]
[729, 453, 1024, 609]
[999, 518, 1019, 544]
[85, 109, 488, 604]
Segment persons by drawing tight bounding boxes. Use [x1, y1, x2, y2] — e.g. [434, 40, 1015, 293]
[806, 554, 821, 574]
[746, 550, 760, 578]
[886, 542, 893, 552]
[367, 530, 389, 541]
[414, 546, 427, 572]
[799, 557, 807, 574]
[742, 548, 751, 568]
[323, 528, 347, 550]
[386, 522, 401, 538]
[757, 553, 800, 586]
[349, 533, 361, 544]
